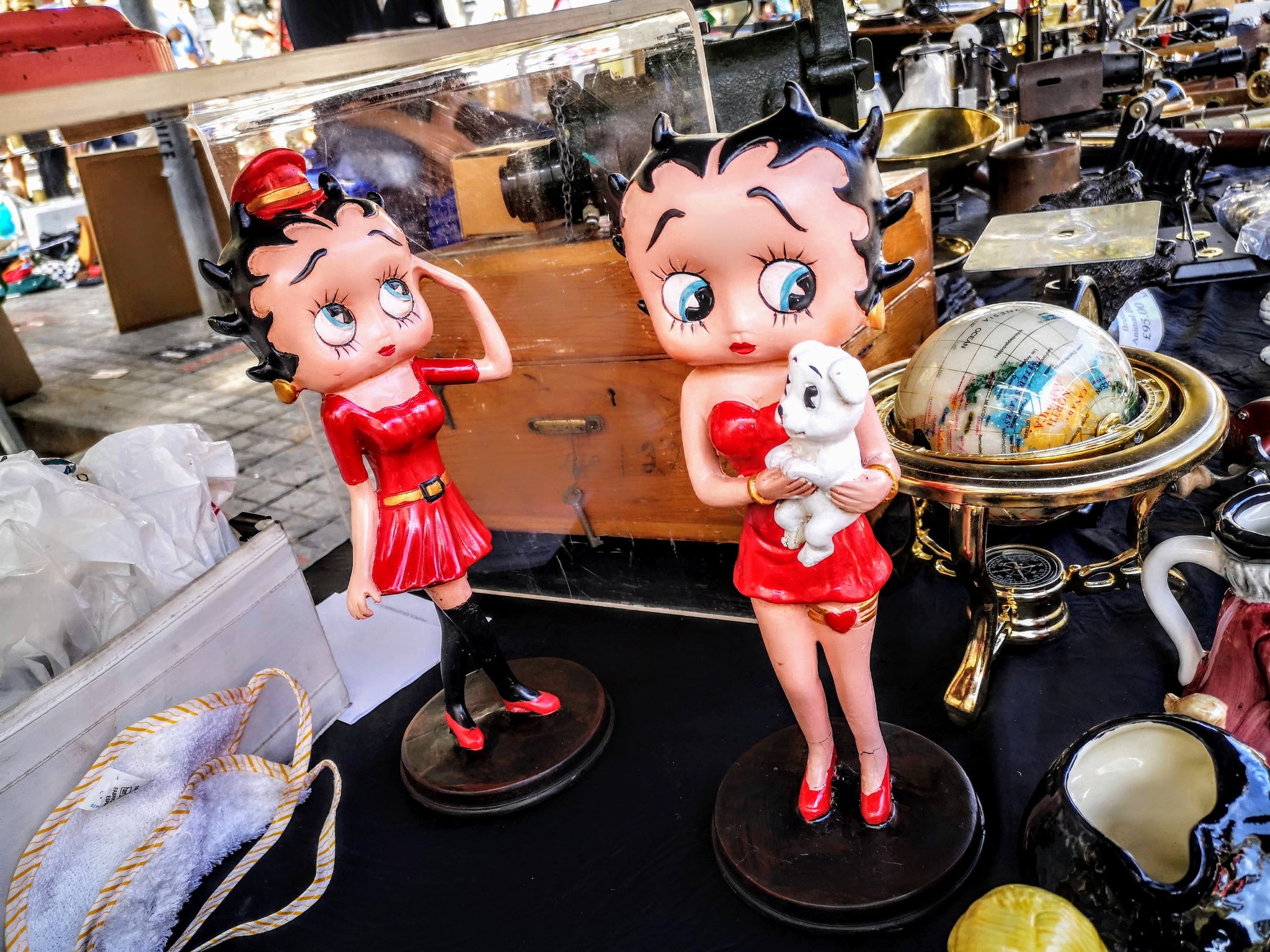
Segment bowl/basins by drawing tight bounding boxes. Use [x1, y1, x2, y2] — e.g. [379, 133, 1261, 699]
[858, 104, 1003, 194]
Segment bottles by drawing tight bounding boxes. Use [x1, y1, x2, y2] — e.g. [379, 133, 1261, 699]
[1020, 710, 1270, 952]
[856, 73, 891, 120]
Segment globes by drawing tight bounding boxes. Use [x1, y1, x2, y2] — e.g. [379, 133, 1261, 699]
[866, 301, 1234, 725]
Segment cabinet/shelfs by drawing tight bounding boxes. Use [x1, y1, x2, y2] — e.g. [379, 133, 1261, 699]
[0, 0, 941, 952]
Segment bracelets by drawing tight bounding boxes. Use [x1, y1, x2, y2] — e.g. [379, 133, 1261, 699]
[745, 476, 779, 508]
[869, 466, 901, 498]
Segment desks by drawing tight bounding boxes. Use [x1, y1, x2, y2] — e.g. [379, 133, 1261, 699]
[854, 2, 1003, 38]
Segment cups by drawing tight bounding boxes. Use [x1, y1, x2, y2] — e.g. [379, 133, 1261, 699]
[1139, 484, 1270, 766]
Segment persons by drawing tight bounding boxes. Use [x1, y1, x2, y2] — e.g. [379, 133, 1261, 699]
[199, 150, 563, 757]
[278, 0, 470, 121]
[608, 82, 917, 831]
